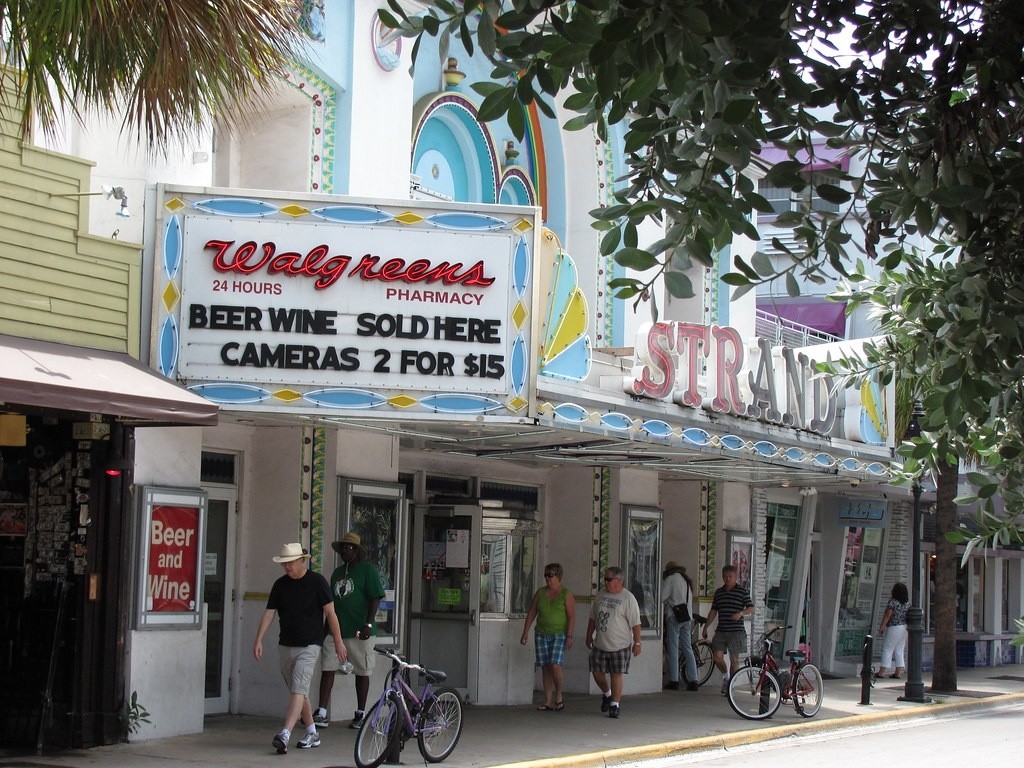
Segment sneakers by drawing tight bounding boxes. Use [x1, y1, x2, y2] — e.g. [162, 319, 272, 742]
[272, 730, 289, 754]
[297, 730, 321, 748]
[601, 693, 611, 712]
[300, 708, 329, 727]
[609, 702, 620, 717]
[349, 711, 365, 729]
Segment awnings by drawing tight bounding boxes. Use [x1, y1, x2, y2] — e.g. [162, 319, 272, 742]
[0, 334, 220, 426]
[756, 303, 847, 337]
[754, 145, 851, 173]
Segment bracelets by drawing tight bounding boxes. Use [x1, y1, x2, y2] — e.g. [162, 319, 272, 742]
[567, 635, 573, 639]
[635, 642, 641, 646]
[740, 612, 743, 617]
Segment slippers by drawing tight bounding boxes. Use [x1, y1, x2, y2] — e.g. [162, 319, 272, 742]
[537, 705, 553, 710]
[889, 674, 901, 678]
[555, 702, 565, 711]
[873, 673, 884, 678]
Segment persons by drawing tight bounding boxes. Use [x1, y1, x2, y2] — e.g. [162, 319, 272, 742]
[702, 566, 755, 698]
[586, 567, 641, 718]
[520, 563, 576, 711]
[873, 583, 912, 679]
[253, 543, 347, 754]
[300, 532, 386, 729]
[660, 562, 699, 691]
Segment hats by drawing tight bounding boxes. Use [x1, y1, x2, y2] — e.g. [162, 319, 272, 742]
[273, 543, 311, 563]
[331, 533, 367, 558]
[663, 562, 685, 580]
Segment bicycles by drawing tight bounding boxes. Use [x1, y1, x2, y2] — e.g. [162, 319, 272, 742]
[353, 644, 464, 768]
[663, 614, 715, 686]
[726, 625, 825, 721]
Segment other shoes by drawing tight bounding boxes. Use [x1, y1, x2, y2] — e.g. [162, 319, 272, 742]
[664, 681, 679, 690]
[687, 681, 698, 691]
[720, 678, 735, 697]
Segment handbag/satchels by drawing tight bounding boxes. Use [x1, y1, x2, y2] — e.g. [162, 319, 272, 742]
[673, 604, 690, 623]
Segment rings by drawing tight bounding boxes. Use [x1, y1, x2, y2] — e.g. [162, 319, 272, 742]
[366, 636, 369, 637]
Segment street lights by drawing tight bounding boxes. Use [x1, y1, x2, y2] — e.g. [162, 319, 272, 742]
[905, 399, 927, 702]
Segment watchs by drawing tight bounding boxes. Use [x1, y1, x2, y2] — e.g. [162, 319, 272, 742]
[365, 623, 372, 628]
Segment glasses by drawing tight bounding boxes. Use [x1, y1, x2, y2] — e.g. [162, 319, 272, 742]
[339, 543, 354, 550]
[604, 577, 619, 582]
[544, 573, 556, 578]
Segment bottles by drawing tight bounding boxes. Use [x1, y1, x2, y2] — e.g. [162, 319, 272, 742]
[335, 653, 354, 674]
